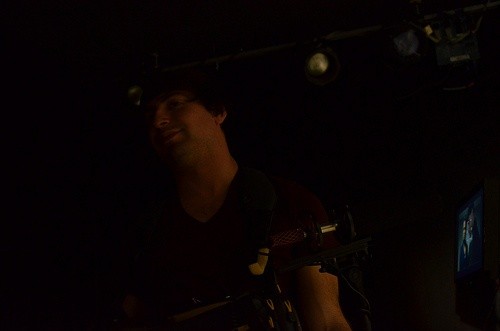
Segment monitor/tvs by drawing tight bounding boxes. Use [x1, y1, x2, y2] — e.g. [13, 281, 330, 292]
[453, 176, 500, 294]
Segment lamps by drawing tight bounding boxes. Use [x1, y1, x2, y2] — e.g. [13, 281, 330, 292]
[304, 38, 329, 75]
[126, 83, 143, 105]
[434, 43, 470, 85]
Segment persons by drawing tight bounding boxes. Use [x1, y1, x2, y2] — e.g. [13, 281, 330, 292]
[458, 201, 482, 272]
[92, 56, 352, 331]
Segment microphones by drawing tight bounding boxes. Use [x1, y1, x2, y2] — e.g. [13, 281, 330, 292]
[256, 179, 276, 255]
